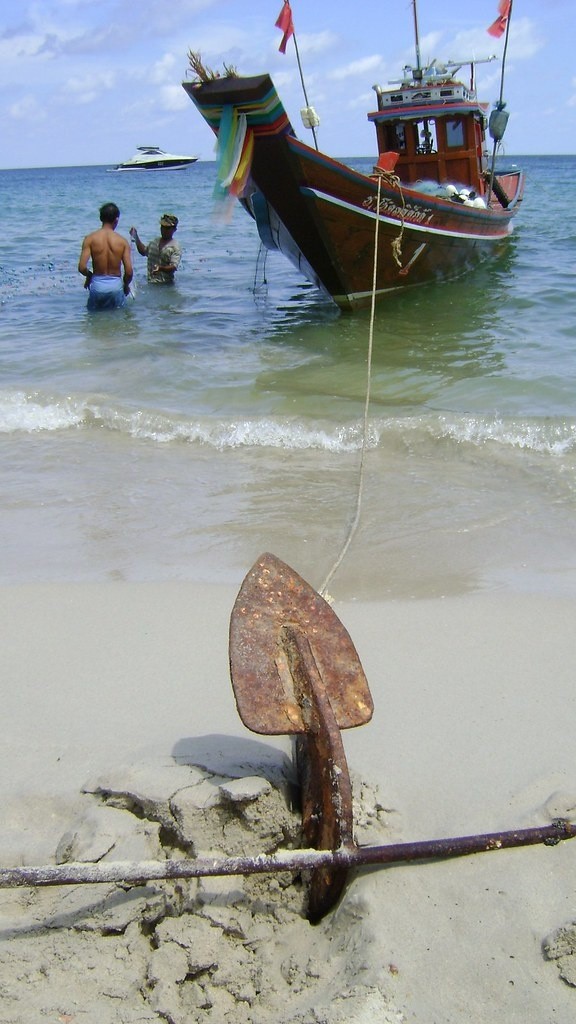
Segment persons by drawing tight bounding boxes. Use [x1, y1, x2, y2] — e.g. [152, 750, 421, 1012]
[78, 203, 134, 308]
[130, 215, 182, 282]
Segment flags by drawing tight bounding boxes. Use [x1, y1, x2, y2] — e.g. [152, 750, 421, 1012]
[275, 2, 295, 54]
[488, 0, 511, 37]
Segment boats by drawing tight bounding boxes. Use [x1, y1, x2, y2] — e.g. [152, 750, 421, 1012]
[182, 0, 526, 316]
[105, 145, 198, 172]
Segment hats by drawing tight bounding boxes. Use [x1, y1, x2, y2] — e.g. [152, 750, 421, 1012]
[159, 214, 178, 226]
